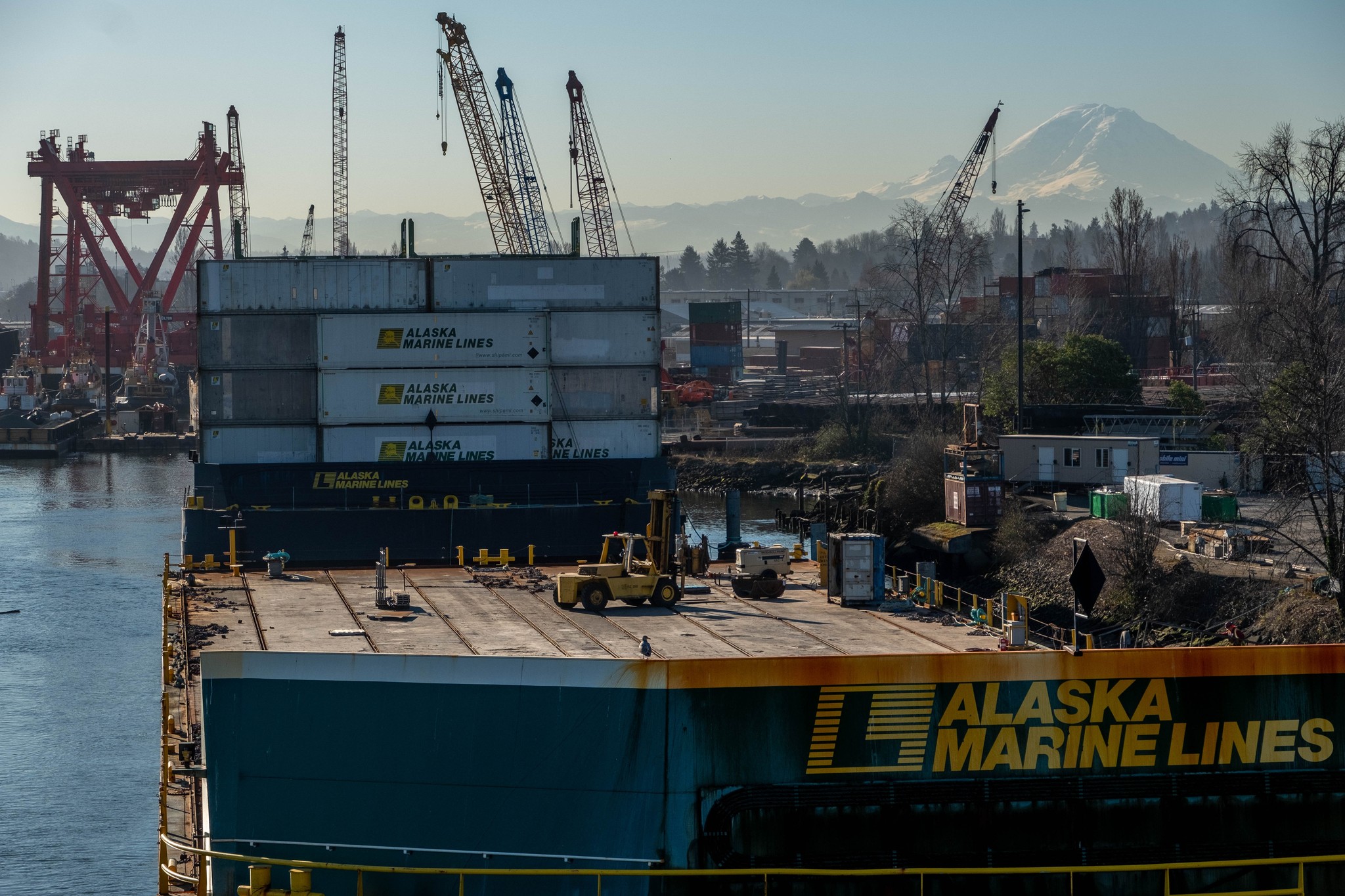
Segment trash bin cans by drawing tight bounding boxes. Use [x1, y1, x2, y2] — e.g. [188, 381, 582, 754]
[1089, 488, 1131, 519]
[1201, 488, 1242, 522]
[1054, 492, 1067, 512]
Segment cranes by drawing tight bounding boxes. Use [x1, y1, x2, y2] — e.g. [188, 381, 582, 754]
[836, 96, 1005, 459]
[435, 11, 637, 257]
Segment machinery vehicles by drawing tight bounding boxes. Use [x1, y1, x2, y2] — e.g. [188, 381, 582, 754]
[553, 489, 726, 611]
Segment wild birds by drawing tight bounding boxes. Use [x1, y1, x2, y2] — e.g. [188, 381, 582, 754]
[638, 636, 652, 659]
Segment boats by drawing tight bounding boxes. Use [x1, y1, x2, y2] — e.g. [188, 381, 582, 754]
[177, 254, 688, 567]
[113, 289, 177, 410]
[158, 531, 1344, 896]
[0, 354, 43, 400]
[58, 346, 103, 398]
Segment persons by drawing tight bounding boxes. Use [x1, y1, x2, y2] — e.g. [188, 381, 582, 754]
[153, 372, 158, 379]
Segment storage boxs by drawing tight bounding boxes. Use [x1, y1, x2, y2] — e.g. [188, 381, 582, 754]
[945, 473, 1005, 526]
[117, 410, 177, 433]
[196, 256, 661, 464]
[751, 346, 842, 376]
[874, 268, 1175, 364]
[668, 301, 744, 380]
